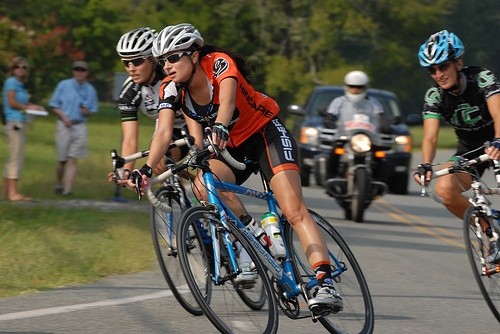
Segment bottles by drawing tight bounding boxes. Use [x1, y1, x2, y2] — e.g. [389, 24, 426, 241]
[238, 214, 267, 247]
[259, 211, 286, 258]
[491, 209, 500, 234]
[191, 194, 212, 237]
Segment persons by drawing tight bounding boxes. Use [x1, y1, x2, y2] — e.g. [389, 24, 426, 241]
[325, 70, 384, 193]
[417, 30, 500, 274]
[3, 56, 47, 201]
[127, 23, 342, 313]
[48, 62, 99, 196]
[108, 26, 258, 284]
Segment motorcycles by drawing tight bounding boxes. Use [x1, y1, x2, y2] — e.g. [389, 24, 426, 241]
[318, 109, 404, 221]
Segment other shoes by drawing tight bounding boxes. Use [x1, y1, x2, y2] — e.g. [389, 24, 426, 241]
[56, 186, 72, 196]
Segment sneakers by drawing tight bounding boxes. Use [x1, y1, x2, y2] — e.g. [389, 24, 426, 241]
[308, 285, 343, 314]
[480, 229, 500, 266]
[232, 262, 258, 282]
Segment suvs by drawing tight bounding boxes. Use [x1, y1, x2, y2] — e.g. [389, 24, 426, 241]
[287, 85, 423, 195]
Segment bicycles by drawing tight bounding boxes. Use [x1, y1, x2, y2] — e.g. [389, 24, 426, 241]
[417, 137, 500, 323]
[111, 127, 268, 316]
[132, 127, 375, 334]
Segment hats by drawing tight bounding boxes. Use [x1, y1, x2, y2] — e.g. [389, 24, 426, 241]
[73, 61, 89, 70]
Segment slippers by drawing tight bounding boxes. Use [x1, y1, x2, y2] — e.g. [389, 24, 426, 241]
[21, 195, 33, 201]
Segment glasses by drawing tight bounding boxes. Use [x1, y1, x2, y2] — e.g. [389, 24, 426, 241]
[426, 62, 451, 75]
[159, 51, 191, 67]
[13, 65, 28, 69]
[122, 53, 152, 67]
[74, 68, 86, 72]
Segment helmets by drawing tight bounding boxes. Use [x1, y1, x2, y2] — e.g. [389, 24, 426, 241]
[116, 26, 157, 60]
[151, 23, 203, 60]
[418, 30, 464, 67]
[344, 70, 369, 102]
